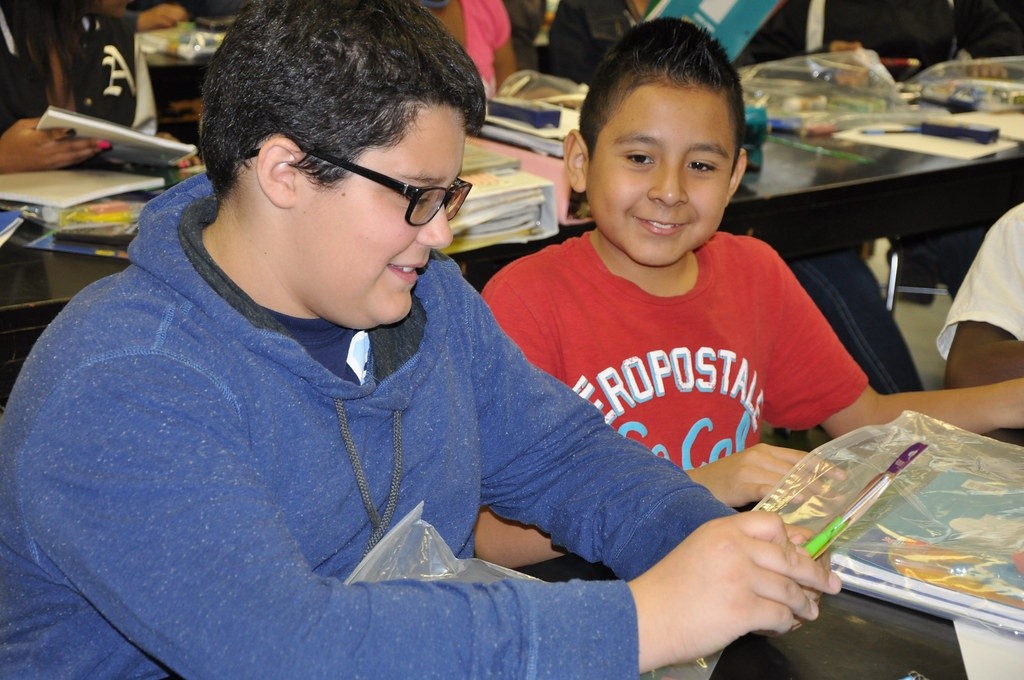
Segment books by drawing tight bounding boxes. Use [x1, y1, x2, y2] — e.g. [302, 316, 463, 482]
[0, 23, 1024, 257]
[828, 458, 1024, 638]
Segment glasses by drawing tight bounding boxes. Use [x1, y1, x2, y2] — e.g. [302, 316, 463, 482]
[243, 136, 472, 227]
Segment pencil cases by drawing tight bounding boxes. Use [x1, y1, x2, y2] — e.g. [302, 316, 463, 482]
[921, 117, 1000, 145]
[489, 97, 560, 128]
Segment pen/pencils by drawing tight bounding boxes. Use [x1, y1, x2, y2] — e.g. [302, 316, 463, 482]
[55, 231, 136, 245]
[803, 475, 888, 560]
[861, 127, 920, 135]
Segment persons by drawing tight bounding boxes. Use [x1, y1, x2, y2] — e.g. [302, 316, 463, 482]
[936, 205, 1024, 446]
[103, 1, 1024, 394]
[0, 1, 202, 175]
[0, 0, 843, 680]
[474, 16, 1024, 568]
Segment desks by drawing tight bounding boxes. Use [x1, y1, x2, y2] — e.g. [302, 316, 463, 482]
[0, 60, 1024, 409]
